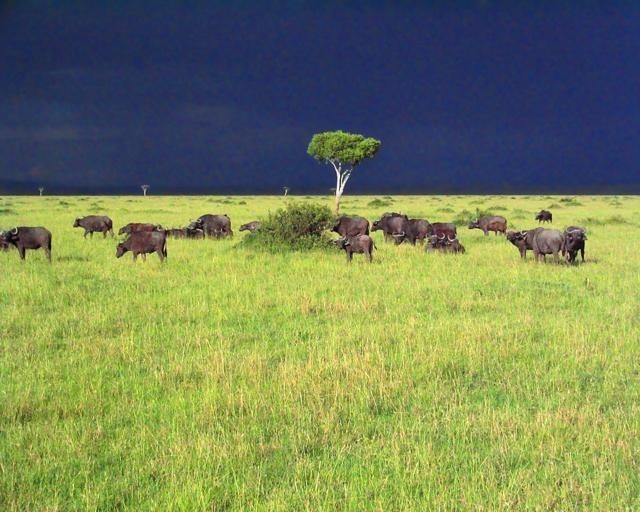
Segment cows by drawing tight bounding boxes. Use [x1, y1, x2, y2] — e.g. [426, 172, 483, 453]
[370, 212, 466, 254]
[118, 213, 259, 240]
[73, 215, 114, 238]
[335, 234, 377, 263]
[116, 231, 167, 263]
[507, 226, 588, 263]
[330, 215, 369, 236]
[535, 210, 552, 223]
[0, 226, 51, 263]
[469, 215, 507, 235]
